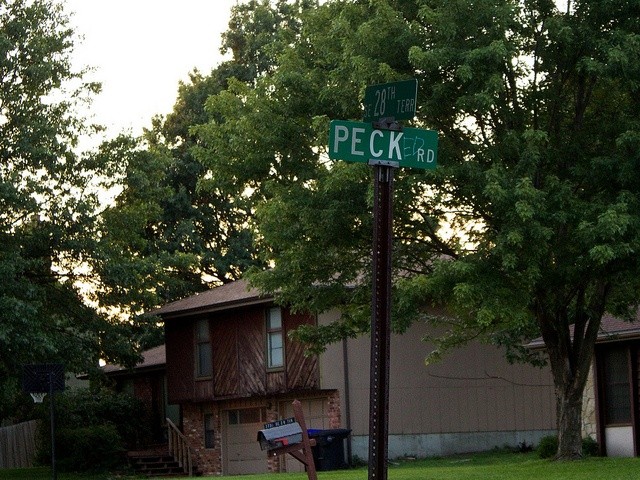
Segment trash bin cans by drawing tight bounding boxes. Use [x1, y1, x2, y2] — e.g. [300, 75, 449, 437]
[309, 429, 351, 471]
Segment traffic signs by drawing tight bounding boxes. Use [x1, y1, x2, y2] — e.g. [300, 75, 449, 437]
[363, 79, 418, 122]
[329, 120, 438, 169]
